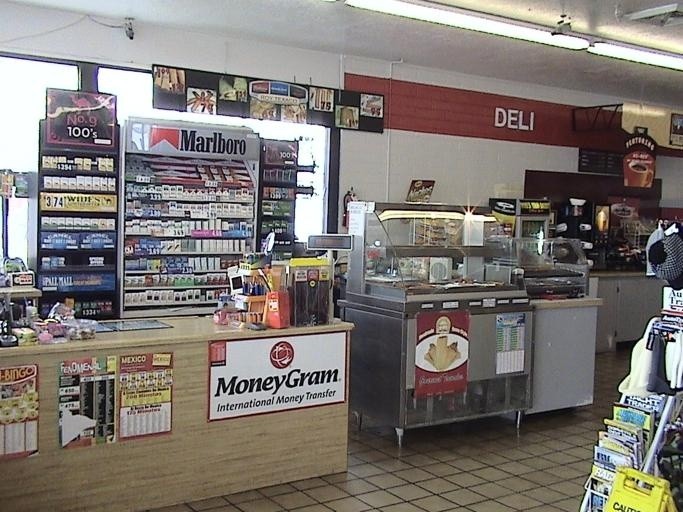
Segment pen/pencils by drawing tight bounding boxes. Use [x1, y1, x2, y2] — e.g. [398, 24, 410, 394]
[243, 278, 265, 296]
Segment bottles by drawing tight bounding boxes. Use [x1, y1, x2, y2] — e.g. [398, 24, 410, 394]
[524, 221, 542, 236]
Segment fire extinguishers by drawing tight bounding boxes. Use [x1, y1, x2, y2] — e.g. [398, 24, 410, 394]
[343, 186, 357, 227]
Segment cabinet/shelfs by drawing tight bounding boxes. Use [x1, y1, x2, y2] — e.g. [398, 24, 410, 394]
[27, 87, 119, 320]
[254, 133, 298, 260]
[119, 116, 261, 319]
[345, 201, 533, 312]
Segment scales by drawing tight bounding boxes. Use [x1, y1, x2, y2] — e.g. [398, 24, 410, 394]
[307, 235, 353, 323]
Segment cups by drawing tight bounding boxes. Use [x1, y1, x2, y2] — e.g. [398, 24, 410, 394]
[629, 162, 654, 187]
[579, 223, 594, 249]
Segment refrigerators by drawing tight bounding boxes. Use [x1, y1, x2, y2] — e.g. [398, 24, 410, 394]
[489, 198, 550, 237]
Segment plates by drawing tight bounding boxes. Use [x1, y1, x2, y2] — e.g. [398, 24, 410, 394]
[415, 334, 469, 373]
